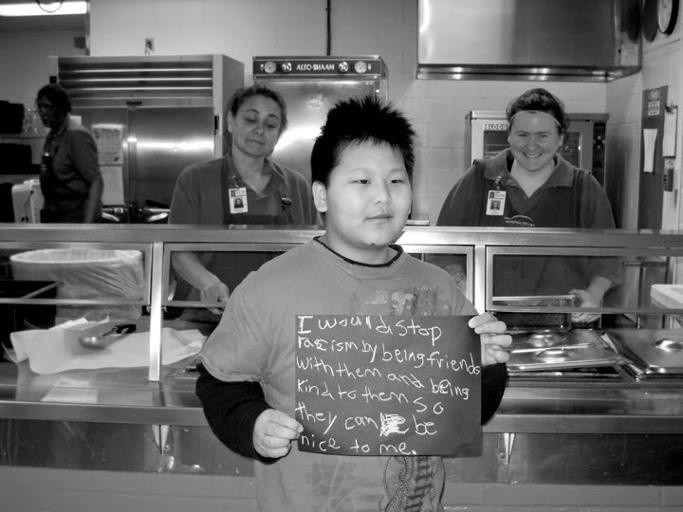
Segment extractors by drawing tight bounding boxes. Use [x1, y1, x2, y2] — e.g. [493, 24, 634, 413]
[409, 1, 644, 80]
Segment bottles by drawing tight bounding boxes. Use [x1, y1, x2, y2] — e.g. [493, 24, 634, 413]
[18, 108, 47, 137]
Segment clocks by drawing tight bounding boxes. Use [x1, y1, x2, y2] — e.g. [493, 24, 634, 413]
[657, 0, 679, 34]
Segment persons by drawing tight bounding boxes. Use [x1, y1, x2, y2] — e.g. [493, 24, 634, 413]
[436, 87, 626, 324]
[196, 92, 514, 512]
[160, 84, 317, 318]
[36, 84, 104, 224]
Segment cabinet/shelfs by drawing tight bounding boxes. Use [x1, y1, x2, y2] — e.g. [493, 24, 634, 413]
[0, 221, 683, 512]
